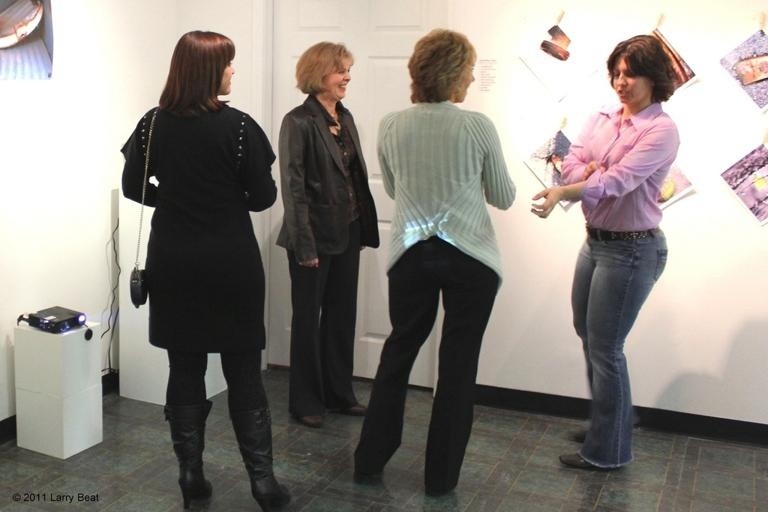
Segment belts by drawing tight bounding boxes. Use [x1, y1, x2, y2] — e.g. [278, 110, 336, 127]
[585, 223, 659, 241]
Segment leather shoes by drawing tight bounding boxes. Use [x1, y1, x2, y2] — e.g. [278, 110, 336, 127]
[575, 429, 588, 442]
[326, 403, 366, 416]
[559, 452, 595, 468]
[303, 415, 323, 428]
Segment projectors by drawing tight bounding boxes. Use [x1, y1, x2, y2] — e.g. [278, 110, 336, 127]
[29, 306, 86, 334]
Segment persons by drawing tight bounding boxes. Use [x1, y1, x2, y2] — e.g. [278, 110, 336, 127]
[275, 42, 380, 428]
[352, 27, 516, 496]
[122, 29, 290, 512]
[531, 35, 679, 471]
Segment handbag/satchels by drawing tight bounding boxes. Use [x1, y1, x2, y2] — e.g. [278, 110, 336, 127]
[130, 268, 148, 307]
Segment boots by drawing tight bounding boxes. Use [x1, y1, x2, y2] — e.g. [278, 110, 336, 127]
[230, 405, 290, 511]
[165, 399, 212, 510]
